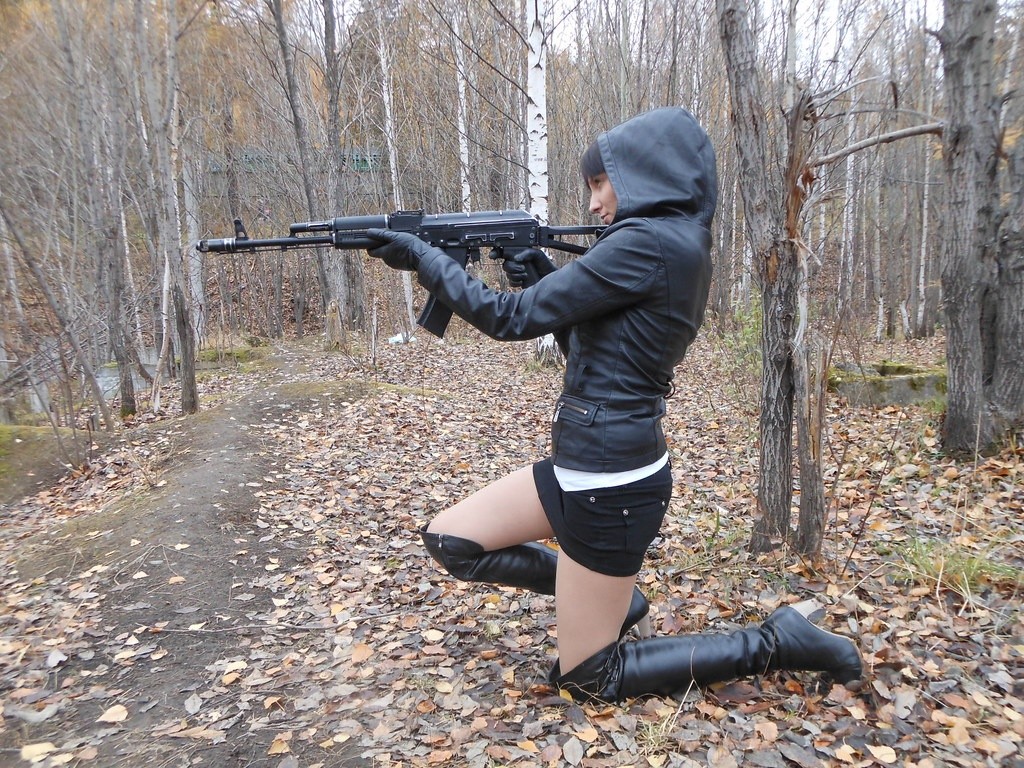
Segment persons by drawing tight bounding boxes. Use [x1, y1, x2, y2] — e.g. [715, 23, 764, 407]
[365, 105, 863, 706]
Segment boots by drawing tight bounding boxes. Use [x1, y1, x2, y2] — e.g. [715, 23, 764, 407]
[547, 595, 866, 706]
[419, 522, 650, 645]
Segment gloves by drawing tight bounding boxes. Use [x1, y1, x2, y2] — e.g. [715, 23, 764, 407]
[489, 245, 559, 287]
[365, 228, 433, 272]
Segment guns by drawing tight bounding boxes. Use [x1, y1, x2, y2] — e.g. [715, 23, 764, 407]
[192, 208, 613, 358]
